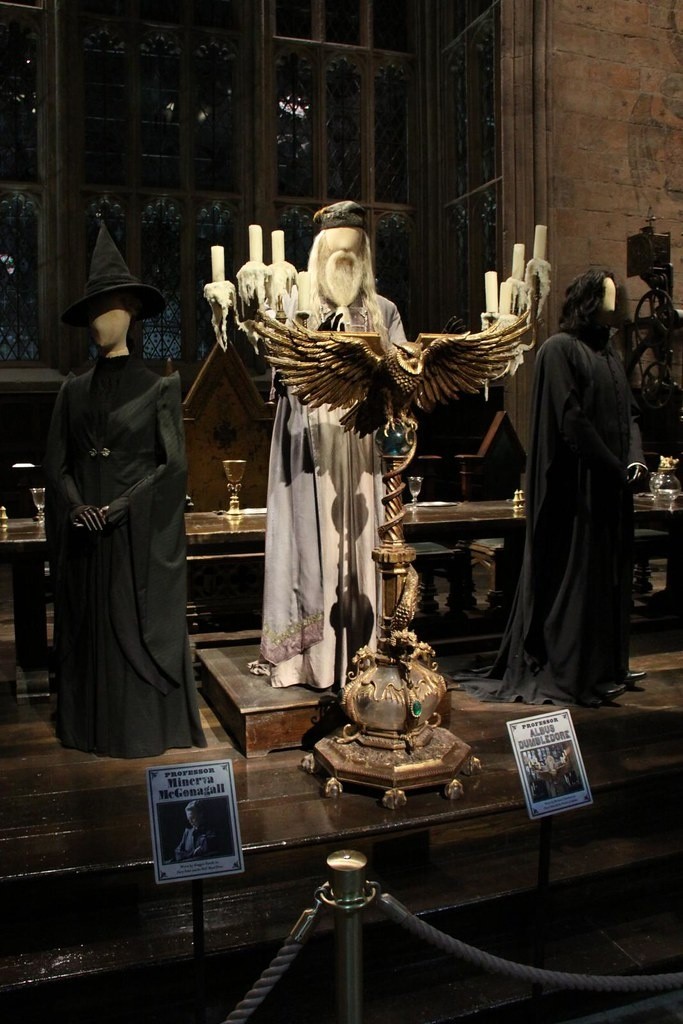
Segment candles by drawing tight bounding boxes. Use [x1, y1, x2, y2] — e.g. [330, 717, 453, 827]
[485, 271, 498, 314]
[249, 224, 263, 262]
[211, 245, 225, 280]
[533, 224, 547, 259]
[512, 243, 525, 282]
[271, 229, 285, 263]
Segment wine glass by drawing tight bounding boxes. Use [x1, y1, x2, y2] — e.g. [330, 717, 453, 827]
[409, 477, 423, 511]
[223, 460, 246, 514]
[30, 488, 45, 525]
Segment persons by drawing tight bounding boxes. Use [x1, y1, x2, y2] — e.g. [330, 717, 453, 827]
[43, 277, 211, 764]
[173, 801, 220, 862]
[243, 200, 409, 691]
[477, 268, 649, 705]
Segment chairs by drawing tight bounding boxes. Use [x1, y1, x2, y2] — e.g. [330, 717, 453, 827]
[454, 410, 527, 592]
[166, 333, 277, 512]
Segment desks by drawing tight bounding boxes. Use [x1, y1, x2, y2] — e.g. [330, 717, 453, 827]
[0, 502, 683, 684]
